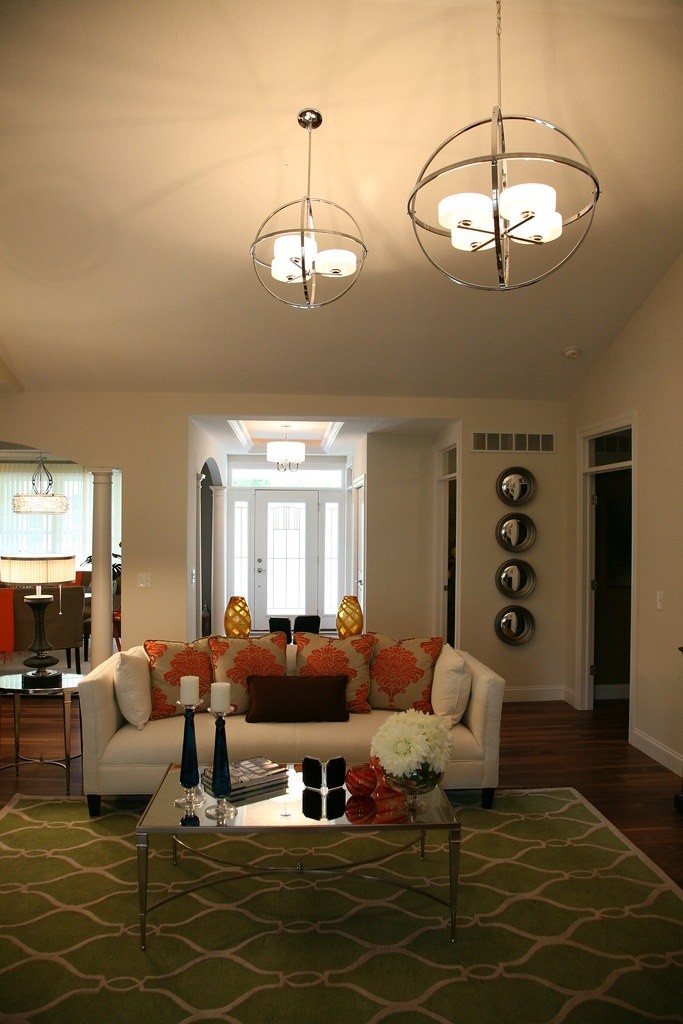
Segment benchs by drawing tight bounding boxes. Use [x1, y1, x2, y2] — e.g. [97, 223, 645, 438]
[0, 571, 121, 669]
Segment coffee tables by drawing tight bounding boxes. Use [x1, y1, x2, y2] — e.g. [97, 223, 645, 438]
[134, 764, 462, 955]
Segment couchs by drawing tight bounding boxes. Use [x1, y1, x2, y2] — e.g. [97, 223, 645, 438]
[79, 645, 506, 818]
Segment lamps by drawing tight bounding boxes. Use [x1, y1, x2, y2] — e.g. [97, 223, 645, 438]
[265, 425, 305, 472]
[11, 449, 70, 515]
[408, 0, 601, 289]
[251, 106, 367, 310]
[0, 553, 76, 689]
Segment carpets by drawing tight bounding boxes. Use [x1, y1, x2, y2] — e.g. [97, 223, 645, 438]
[0, 786, 683, 1024]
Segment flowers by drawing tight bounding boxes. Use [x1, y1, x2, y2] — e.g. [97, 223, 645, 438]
[368, 708, 453, 778]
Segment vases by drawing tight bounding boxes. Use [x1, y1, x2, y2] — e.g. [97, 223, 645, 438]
[381, 759, 444, 816]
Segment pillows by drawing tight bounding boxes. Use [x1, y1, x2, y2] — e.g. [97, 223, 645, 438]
[113, 631, 472, 729]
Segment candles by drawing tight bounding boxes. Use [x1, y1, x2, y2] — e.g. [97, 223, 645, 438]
[210, 681, 230, 711]
[181, 673, 199, 705]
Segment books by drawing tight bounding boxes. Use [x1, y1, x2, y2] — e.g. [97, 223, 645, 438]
[201, 755, 289, 802]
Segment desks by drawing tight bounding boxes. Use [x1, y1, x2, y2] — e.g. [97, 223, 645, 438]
[0, 673, 85, 796]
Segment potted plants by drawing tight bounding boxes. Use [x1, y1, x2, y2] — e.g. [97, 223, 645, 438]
[80, 551, 122, 596]
